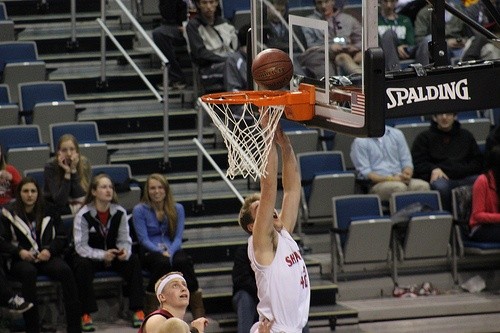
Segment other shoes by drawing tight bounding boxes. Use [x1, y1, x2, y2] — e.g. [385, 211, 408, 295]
[156, 78, 188, 91]
[130, 310, 145, 327]
[81, 314, 96, 332]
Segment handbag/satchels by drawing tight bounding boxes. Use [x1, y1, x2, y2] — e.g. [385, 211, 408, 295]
[390, 201, 439, 245]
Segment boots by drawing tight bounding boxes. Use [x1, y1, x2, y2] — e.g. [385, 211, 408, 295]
[143, 291, 158, 316]
[189, 291, 209, 331]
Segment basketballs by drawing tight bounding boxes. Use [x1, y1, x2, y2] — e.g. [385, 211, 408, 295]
[251, 48, 294, 90]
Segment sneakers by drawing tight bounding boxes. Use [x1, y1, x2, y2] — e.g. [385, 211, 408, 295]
[0, 294, 34, 314]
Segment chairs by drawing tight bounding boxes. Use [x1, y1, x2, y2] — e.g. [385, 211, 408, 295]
[0, 0, 500, 333]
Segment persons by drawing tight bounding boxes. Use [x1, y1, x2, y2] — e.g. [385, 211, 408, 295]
[229, 107, 311, 333]
[468, 123, 500, 244]
[418, 113, 483, 212]
[0, 134, 92, 333]
[73, 174, 145, 332]
[152, 0, 500, 93]
[138, 272, 209, 333]
[132, 174, 206, 320]
[350, 124, 431, 202]
